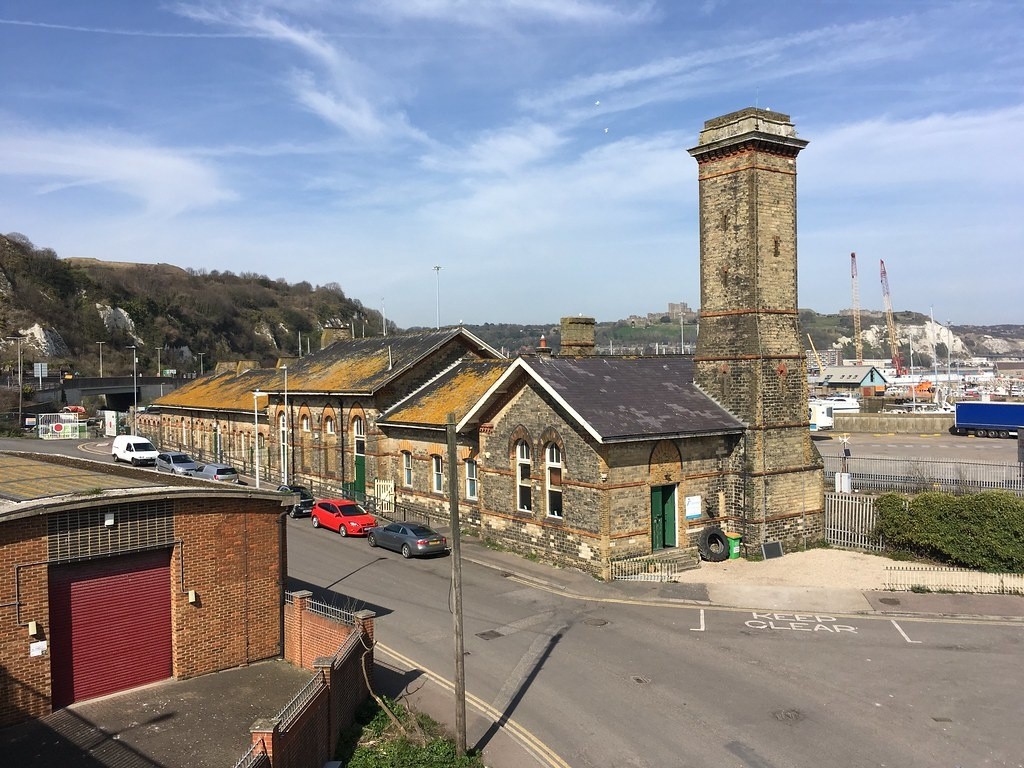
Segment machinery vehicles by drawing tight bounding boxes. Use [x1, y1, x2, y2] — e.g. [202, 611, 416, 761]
[806, 333, 823, 377]
[851, 252, 862, 366]
[880, 259, 905, 378]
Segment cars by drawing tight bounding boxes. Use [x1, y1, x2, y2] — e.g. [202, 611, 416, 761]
[155, 452, 198, 475]
[311, 499, 378, 537]
[277, 485, 316, 518]
[368, 521, 446, 559]
[189, 463, 239, 483]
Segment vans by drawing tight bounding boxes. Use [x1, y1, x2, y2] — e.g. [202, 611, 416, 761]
[112, 435, 160, 467]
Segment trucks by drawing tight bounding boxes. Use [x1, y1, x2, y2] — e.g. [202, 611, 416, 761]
[955, 402, 1024, 439]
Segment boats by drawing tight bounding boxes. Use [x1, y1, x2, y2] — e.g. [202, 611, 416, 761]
[817, 396, 861, 410]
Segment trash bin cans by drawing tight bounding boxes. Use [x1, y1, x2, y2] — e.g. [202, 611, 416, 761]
[725, 533, 743, 559]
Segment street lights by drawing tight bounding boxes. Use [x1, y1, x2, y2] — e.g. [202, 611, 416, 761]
[198, 353, 206, 376]
[432, 264, 445, 330]
[280, 364, 287, 485]
[945, 319, 955, 393]
[126, 346, 137, 436]
[6, 337, 26, 386]
[96, 342, 105, 378]
[252, 392, 267, 488]
[679, 312, 686, 355]
[155, 347, 163, 377]
